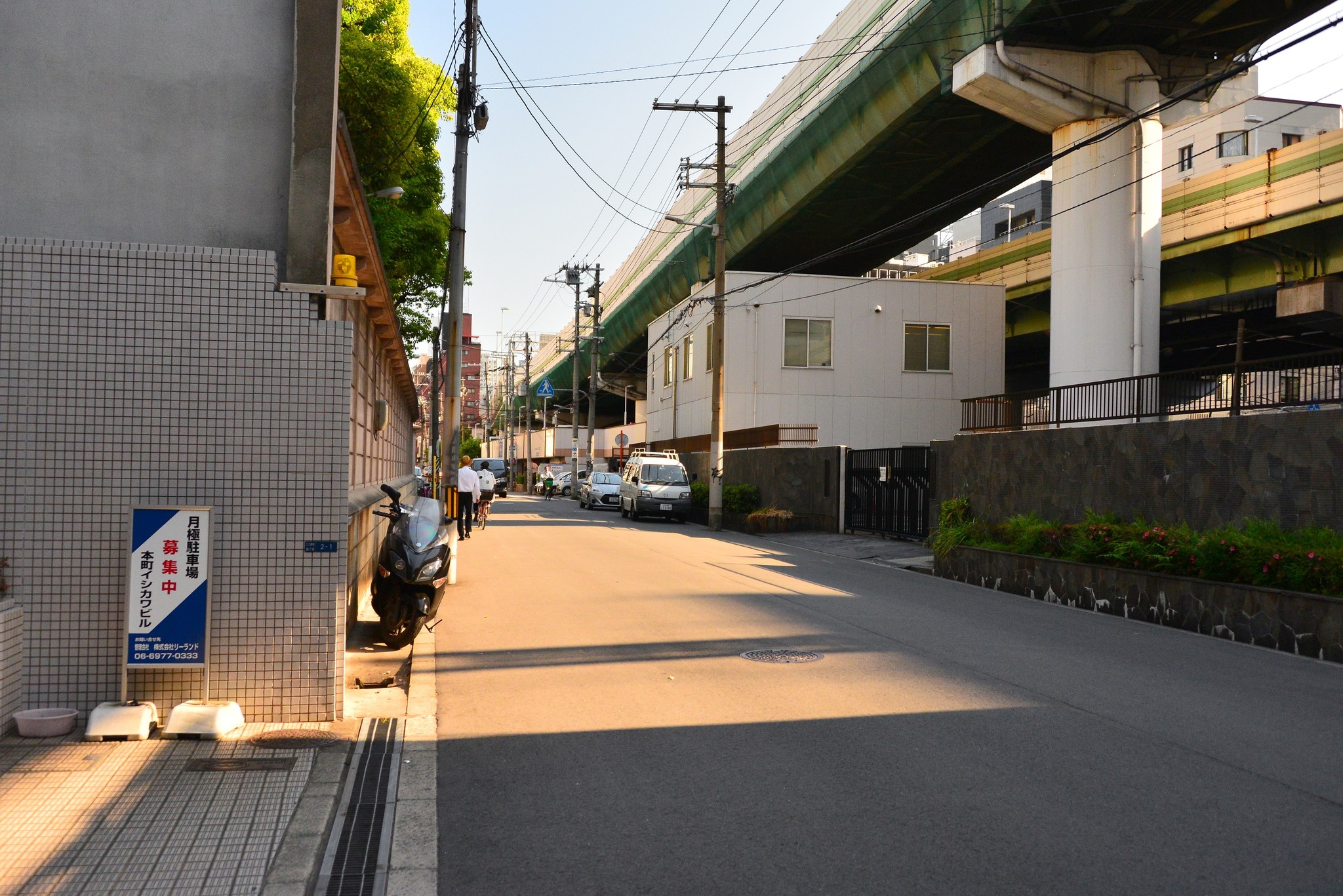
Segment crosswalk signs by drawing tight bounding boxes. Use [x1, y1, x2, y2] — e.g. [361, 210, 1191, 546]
[537, 377, 554, 397]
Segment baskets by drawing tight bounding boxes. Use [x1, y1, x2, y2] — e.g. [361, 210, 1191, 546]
[418, 482, 424, 487]
[545, 481, 553, 486]
[479, 489, 494, 501]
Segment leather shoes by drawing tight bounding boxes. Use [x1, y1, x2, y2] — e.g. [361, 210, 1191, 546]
[549, 496, 553, 498]
[459, 537, 464, 541]
[541, 492, 545, 496]
[465, 533, 471, 538]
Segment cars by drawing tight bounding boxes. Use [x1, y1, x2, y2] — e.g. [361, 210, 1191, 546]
[579, 472, 622, 511]
[556, 470, 587, 496]
[533, 471, 572, 495]
[414, 466, 443, 485]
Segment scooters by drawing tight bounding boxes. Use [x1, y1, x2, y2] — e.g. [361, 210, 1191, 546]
[370, 484, 452, 650]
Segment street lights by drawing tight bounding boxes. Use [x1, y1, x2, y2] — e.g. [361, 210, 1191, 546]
[554, 404, 578, 500]
[662, 215, 725, 533]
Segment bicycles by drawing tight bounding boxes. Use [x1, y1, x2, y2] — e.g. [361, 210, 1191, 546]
[542, 477, 554, 502]
[477, 489, 495, 530]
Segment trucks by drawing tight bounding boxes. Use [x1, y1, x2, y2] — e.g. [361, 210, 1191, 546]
[470, 458, 508, 498]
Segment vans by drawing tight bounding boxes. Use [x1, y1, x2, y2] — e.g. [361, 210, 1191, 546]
[619, 447, 693, 524]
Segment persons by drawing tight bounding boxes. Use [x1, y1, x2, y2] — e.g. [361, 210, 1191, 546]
[611, 466, 617, 473]
[457, 455, 481, 542]
[473, 461, 496, 522]
[541, 466, 554, 495]
